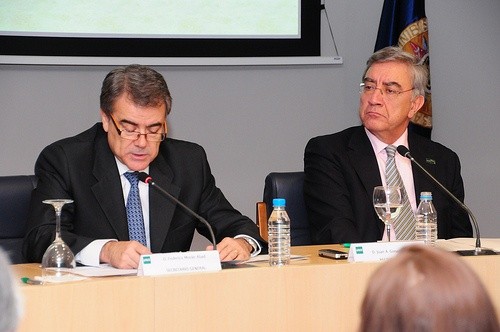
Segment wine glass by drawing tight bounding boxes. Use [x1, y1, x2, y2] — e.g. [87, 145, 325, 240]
[372, 186, 402, 242]
[41, 199, 76, 281]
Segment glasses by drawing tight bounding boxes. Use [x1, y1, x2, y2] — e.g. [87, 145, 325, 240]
[359, 82, 414, 99]
[107, 112, 167, 142]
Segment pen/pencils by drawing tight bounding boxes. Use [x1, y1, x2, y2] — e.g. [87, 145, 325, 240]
[22, 278, 43, 285]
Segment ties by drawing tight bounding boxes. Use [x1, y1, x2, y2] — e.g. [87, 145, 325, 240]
[123, 170, 147, 252]
[384, 145, 417, 240]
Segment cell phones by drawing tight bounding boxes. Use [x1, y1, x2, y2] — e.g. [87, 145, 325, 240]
[319, 249, 348, 259]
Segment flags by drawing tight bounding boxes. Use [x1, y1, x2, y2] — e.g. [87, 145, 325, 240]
[373, 0, 432, 137]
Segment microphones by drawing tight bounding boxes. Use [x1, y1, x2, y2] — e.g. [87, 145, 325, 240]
[137, 172, 217, 250]
[396, 145, 499, 256]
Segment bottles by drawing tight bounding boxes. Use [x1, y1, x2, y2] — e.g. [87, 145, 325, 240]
[268, 198, 291, 266]
[415, 192, 437, 248]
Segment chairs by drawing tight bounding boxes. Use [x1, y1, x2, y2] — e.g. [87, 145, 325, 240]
[256, 171, 311, 246]
[0, 175, 38, 264]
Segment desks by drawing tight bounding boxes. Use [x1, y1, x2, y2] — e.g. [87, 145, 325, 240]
[9, 238, 500, 332]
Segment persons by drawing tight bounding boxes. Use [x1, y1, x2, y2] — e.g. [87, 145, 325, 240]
[303, 47, 474, 244]
[357, 245, 500, 332]
[24, 65, 269, 270]
